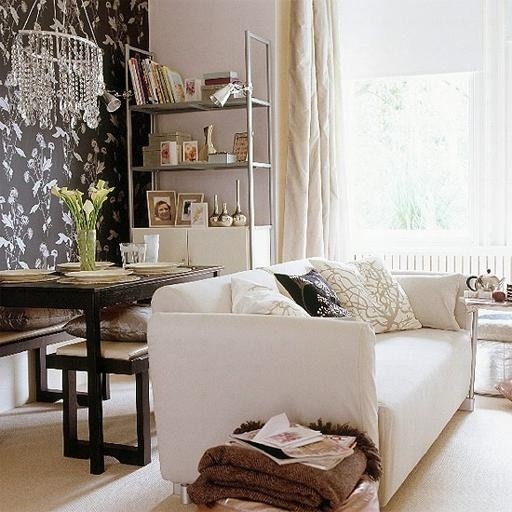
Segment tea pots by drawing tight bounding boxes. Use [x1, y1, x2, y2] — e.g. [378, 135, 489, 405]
[466, 268, 506, 299]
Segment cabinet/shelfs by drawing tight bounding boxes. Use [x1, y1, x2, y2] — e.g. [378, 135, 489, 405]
[132, 228, 272, 270]
[125, 31, 272, 228]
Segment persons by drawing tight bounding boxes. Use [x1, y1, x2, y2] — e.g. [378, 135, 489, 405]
[161, 143, 169, 162]
[185, 143, 196, 160]
[155, 201, 171, 220]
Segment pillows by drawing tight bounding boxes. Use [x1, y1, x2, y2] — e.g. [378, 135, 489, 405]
[0, 305, 77, 331]
[229, 258, 462, 334]
[66, 302, 151, 342]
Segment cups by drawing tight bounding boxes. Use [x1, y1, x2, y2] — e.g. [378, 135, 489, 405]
[119, 242, 146, 268]
[144, 234, 160, 263]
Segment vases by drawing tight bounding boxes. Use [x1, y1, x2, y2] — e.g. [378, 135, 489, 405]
[77, 230, 98, 271]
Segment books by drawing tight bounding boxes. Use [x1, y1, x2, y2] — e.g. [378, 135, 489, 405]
[463, 288, 506, 306]
[232, 411, 359, 471]
[202, 72, 242, 85]
[128, 57, 186, 105]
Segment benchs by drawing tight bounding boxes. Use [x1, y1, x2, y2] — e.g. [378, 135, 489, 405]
[47, 340, 152, 466]
[0, 323, 111, 403]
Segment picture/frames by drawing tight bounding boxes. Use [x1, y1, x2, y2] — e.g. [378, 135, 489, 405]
[178, 193, 204, 225]
[184, 142, 198, 163]
[233, 132, 251, 162]
[147, 191, 178, 227]
[191, 203, 208, 228]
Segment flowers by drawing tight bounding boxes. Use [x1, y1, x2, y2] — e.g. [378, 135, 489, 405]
[50, 180, 114, 271]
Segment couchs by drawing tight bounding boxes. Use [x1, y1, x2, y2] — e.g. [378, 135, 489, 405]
[149, 257, 478, 507]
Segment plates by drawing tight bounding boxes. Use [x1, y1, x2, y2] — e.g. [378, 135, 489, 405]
[62, 269, 134, 282]
[57, 261, 115, 272]
[0, 268, 57, 280]
[125, 262, 184, 273]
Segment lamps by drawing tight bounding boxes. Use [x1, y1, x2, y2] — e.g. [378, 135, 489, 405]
[11, 0, 121, 129]
